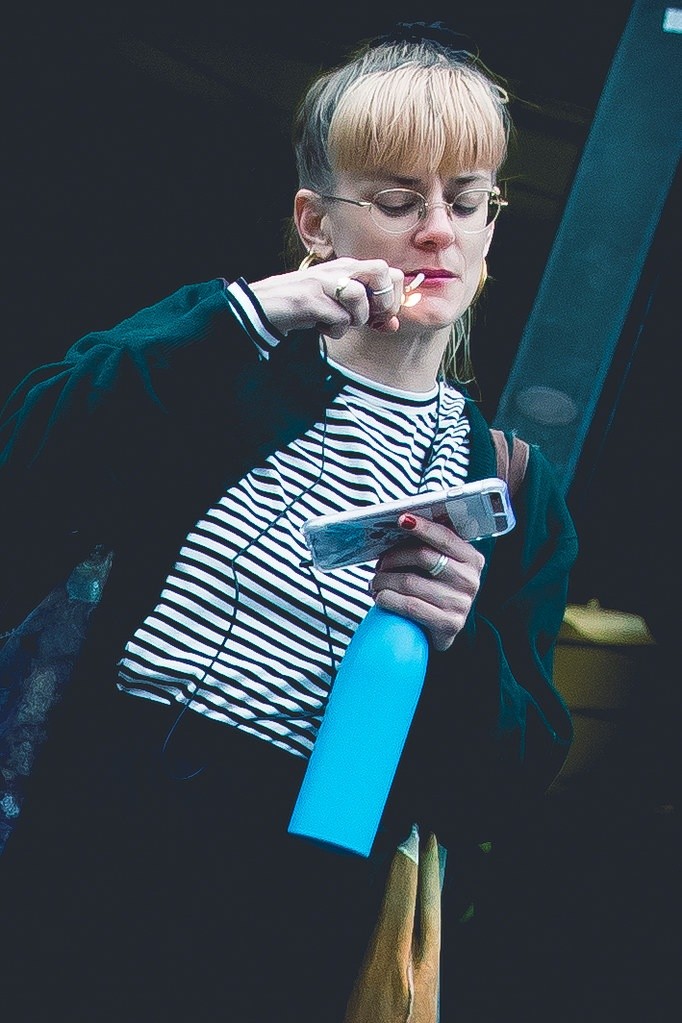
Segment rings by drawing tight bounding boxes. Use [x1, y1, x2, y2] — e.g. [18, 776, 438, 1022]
[371, 283, 394, 294]
[427, 554, 450, 577]
[331, 279, 352, 301]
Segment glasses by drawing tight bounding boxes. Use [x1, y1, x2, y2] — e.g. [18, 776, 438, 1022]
[324, 186, 510, 234]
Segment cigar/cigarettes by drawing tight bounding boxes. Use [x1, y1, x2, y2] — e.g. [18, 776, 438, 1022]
[405, 272, 425, 293]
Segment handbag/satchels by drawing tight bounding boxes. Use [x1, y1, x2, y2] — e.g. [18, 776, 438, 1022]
[343, 821, 441, 1023]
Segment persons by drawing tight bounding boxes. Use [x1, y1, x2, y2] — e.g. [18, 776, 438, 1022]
[0, 42, 577, 1023]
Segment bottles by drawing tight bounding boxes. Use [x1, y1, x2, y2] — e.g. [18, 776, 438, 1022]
[287, 603, 428, 858]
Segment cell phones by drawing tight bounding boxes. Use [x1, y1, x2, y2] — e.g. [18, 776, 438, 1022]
[302, 479, 517, 574]
